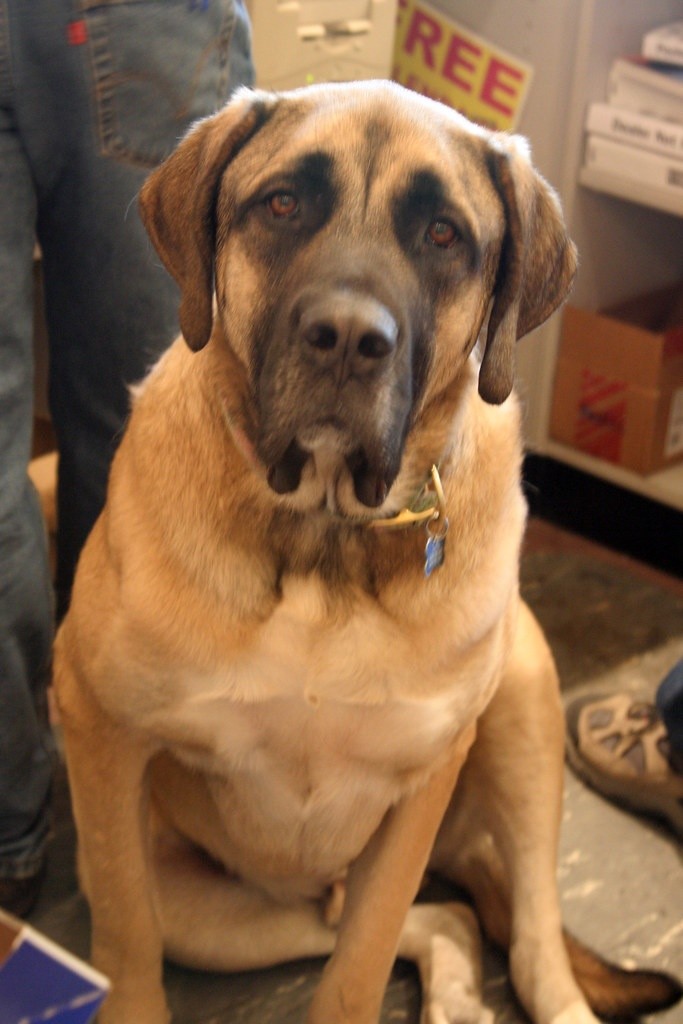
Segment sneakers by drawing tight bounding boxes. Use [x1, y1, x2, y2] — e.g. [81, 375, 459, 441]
[563, 692, 683, 831]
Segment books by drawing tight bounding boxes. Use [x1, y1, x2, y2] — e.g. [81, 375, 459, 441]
[585, 22, 683, 194]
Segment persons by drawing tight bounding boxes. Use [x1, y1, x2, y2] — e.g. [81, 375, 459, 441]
[563, 656, 683, 833]
[0, 0, 255, 919]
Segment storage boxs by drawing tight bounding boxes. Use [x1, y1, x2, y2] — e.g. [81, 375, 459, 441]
[549, 288, 683, 474]
[584, 24, 683, 189]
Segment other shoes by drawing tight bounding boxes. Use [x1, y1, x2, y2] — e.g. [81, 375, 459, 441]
[0, 856, 54, 919]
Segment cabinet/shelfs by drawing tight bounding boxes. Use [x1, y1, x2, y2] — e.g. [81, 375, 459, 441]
[524, 0, 683, 513]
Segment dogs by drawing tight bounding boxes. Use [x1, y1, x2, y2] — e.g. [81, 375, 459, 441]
[53, 78, 602, 1024]
